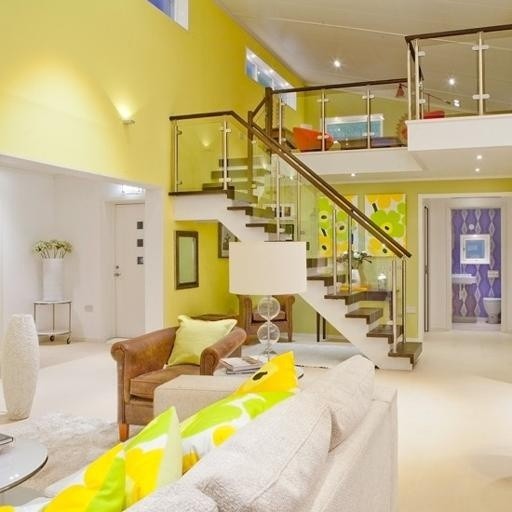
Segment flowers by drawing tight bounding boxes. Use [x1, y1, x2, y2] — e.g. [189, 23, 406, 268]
[335, 249, 374, 271]
[33, 237, 74, 260]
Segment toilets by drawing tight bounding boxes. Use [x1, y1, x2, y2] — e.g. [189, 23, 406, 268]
[483, 297, 501, 323]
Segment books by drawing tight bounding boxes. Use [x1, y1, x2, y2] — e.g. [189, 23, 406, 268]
[219, 354, 303, 379]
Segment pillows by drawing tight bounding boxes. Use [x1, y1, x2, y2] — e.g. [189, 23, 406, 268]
[165, 313, 238, 368]
[42, 448, 128, 510]
[176, 390, 294, 476]
[249, 295, 266, 310]
[236, 349, 300, 416]
[84, 406, 184, 506]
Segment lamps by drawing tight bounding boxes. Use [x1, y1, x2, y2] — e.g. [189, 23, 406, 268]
[227, 240, 307, 360]
[395, 83, 451, 112]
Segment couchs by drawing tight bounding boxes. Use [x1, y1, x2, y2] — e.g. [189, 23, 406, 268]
[23, 354, 399, 512]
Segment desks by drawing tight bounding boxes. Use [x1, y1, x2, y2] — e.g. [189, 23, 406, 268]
[262, 127, 295, 149]
[1, 434, 50, 493]
[315, 288, 399, 343]
[337, 136, 403, 150]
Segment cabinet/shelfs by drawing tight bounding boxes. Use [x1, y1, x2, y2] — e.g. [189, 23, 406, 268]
[33, 300, 73, 346]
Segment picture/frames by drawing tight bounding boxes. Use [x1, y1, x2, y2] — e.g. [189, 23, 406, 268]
[218, 223, 237, 259]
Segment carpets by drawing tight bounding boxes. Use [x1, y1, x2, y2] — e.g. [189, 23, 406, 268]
[2, 408, 147, 511]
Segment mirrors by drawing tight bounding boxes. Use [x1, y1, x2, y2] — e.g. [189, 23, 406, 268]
[174, 229, 200, 291]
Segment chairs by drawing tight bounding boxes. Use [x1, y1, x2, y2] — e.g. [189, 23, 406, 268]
[111, 319, 246, 442]
[293, 127, 332, 151]
[237, 294, 295, 345]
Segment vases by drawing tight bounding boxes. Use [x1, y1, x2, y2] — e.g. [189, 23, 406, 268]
[41, 257, 65, 301]
[351, 268, 360, 285]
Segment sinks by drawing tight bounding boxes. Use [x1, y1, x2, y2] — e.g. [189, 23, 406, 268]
[451, 276, 476, 284]
